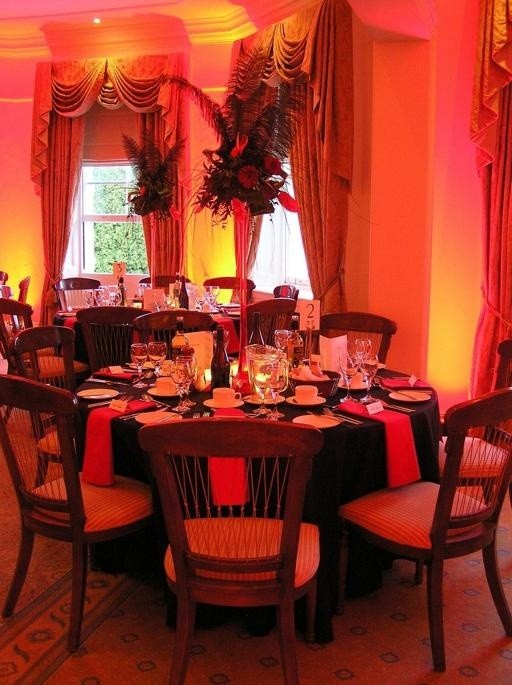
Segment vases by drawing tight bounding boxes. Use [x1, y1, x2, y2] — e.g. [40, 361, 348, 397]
[234, 205, 250, 394]
[148, 209, 159, 289]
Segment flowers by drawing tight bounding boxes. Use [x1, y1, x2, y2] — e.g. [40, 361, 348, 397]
[158, 51, 309, 375]
[119, 106, 186, 218]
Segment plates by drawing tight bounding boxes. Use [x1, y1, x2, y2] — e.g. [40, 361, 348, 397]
[135, 410, 183, 424]
[293, 415, 341, 428]
[77, 389, 118, 399]
[389, 391, 431, 404]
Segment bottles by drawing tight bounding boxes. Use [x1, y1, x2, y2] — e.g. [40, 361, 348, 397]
[174, 272, 188, 311]
[247, 312, 266, 346]
[211, 323, 231, 387]
[288, 315, 305, 366]
[171, 316, 190, 357]
[117, 276, 128, 308]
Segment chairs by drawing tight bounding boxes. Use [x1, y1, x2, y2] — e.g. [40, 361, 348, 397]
[1, 375, 175, 653]
[1, 272, 510, 642]
[335, 386, 510, 673]
[139, 419, 322, 684]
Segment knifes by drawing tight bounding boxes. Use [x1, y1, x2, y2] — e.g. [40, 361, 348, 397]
[382, 386, 418, 401]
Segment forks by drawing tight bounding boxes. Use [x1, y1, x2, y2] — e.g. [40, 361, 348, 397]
[86, 394, 133, 410]
[371, 398, 416, 415]
[322, 407, 365, 426]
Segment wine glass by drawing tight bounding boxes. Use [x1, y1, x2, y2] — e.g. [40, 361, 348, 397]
[130, 329, 381, 419]
[83, 283, 243, 319]
